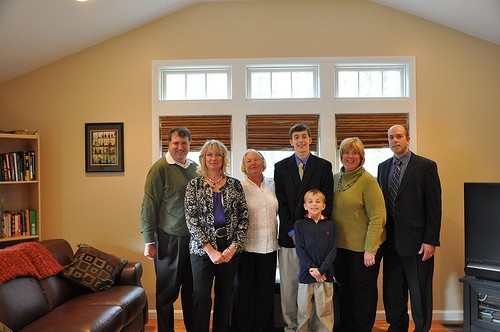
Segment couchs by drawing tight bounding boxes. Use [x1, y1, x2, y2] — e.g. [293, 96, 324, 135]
[0, 239, 149, 332]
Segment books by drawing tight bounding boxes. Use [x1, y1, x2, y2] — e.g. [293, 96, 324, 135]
[0, 209, 37, 238]
[0, 149, 38, 182]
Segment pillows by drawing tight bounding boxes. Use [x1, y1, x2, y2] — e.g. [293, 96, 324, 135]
[61, 243, 127, 291]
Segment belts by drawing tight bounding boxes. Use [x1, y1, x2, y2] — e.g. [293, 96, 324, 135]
[215, 227, 227, 238]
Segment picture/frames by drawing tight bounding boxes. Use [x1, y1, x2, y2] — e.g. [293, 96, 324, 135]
[85, 123, 124, 172]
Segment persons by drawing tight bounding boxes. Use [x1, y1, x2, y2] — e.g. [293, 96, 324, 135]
[184, 139, 249, 332]
[332, 136, 387, 332]
[140, 125, 203, 332]
[294, 189, 339, 332]
[376, 123, 442, 332]
[229, 149, 280, 332]
[274, 124, 335, 332]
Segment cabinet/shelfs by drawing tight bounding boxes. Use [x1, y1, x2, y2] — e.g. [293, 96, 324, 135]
[0, 130, 41, 241]
[458, 275, 500, 332]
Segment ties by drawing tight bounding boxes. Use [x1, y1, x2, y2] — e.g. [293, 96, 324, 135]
[388, 160, 401, 219]
[298, 162, 304, 182]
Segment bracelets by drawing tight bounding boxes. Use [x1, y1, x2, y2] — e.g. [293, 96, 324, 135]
[227, 248, 233, 256]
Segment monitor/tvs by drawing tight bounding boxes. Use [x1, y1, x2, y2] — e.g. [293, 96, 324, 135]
[463, 182, 500, 282]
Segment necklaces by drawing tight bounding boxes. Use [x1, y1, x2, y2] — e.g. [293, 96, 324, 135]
[337, 167, 365, 192]
[204, 173, 222, 189]
